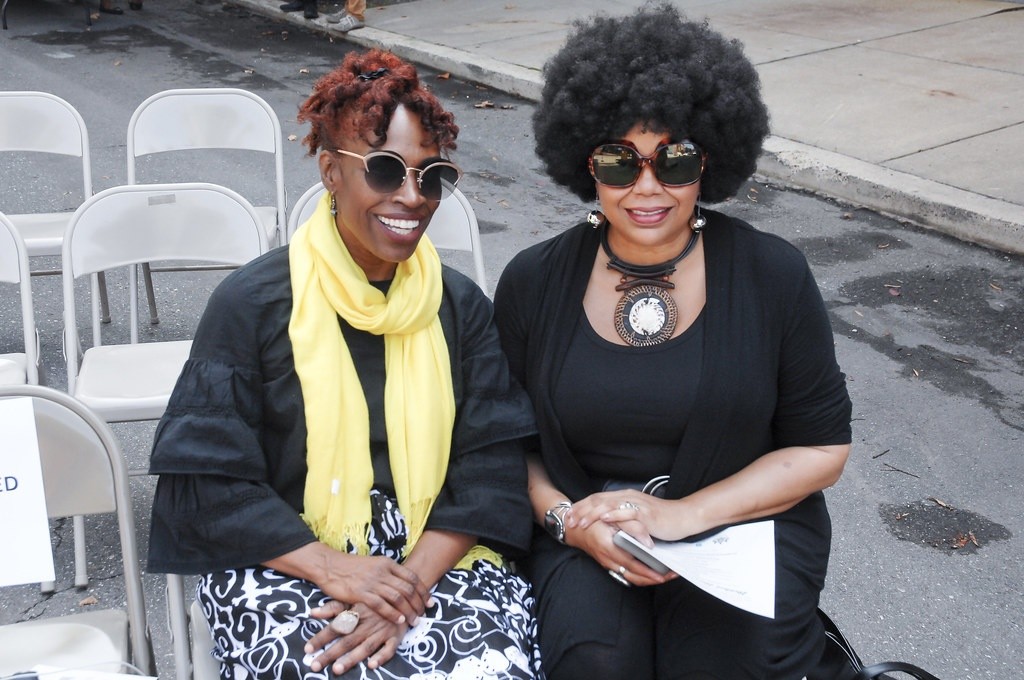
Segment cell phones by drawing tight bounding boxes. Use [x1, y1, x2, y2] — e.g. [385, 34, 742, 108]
[613, 530, 671, 576]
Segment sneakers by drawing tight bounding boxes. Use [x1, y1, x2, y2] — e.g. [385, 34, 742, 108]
[331, 14, 365, 32]
[326, 8, 348, 23]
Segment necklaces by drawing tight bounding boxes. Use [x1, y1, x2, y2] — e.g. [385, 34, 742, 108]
[601, 214, 701, 347]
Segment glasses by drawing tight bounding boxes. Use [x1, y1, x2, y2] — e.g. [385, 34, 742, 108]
[325, 147, 464, 201]
[587, 137, 708, 188]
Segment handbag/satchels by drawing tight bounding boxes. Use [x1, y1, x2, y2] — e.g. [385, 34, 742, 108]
[806, 607, 941, 680]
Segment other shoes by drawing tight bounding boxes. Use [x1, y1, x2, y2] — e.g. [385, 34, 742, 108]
[98, 6, 123, 15]
[280, 0, 304, 11]
[303, 3, 319, 19]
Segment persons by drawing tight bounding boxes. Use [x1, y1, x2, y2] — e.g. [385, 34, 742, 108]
[326, 0, 366, 32]
[280, 0, 318, 18]
[492, 0, 852, 680]
[99, 0, 142, 15]
[147, 48, 545, 680]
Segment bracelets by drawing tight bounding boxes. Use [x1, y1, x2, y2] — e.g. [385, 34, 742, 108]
[544, 501, 574, 545]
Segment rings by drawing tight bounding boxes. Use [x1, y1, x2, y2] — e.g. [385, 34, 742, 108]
[332, 609, 360, 634]
[608, 566, 630, 586]
[619, 501, 640, 519]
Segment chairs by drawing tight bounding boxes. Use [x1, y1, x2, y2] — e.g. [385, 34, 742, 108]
[0, 86, 487, 680]
